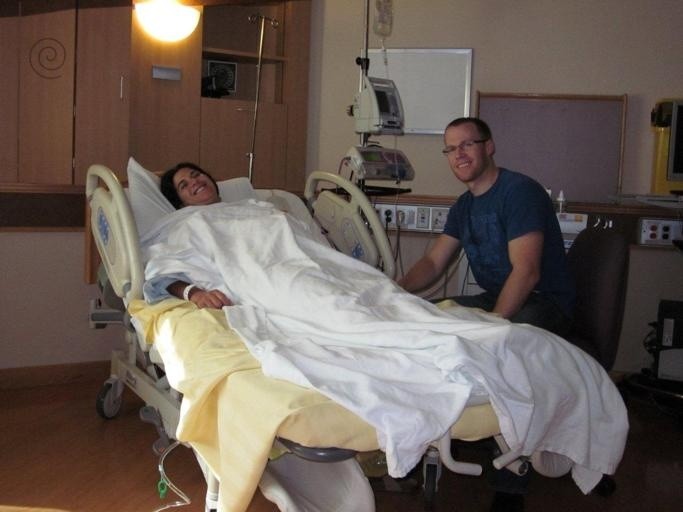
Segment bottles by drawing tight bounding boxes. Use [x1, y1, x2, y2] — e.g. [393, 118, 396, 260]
[556, 190, 567, 212]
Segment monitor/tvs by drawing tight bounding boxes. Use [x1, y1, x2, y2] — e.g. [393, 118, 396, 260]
[666, 98, 683, 181]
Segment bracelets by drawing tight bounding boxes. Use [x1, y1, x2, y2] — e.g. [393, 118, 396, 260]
[180, 284, 196, 301]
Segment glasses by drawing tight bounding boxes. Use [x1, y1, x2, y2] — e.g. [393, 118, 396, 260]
[442, 139, 488, 156]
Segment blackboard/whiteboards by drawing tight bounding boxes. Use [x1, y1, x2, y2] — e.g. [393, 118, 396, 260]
[355, 48, 472, 135]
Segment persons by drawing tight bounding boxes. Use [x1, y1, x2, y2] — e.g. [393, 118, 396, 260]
[390, 113, 585, 494]
[138, 155, 233, 310]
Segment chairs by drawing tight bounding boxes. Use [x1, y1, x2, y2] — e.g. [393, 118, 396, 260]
[565, 232, 630, 373]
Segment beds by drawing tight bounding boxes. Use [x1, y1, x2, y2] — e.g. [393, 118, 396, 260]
[84, 165, 603, 511]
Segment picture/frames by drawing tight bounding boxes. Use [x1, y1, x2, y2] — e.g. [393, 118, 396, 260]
[475, 88, 628, 207]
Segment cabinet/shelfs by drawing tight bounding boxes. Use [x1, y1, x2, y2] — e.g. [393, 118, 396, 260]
[0, 0, 310, 195]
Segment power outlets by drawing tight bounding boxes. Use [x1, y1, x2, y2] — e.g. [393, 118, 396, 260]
[373, 203, 453, 233]
